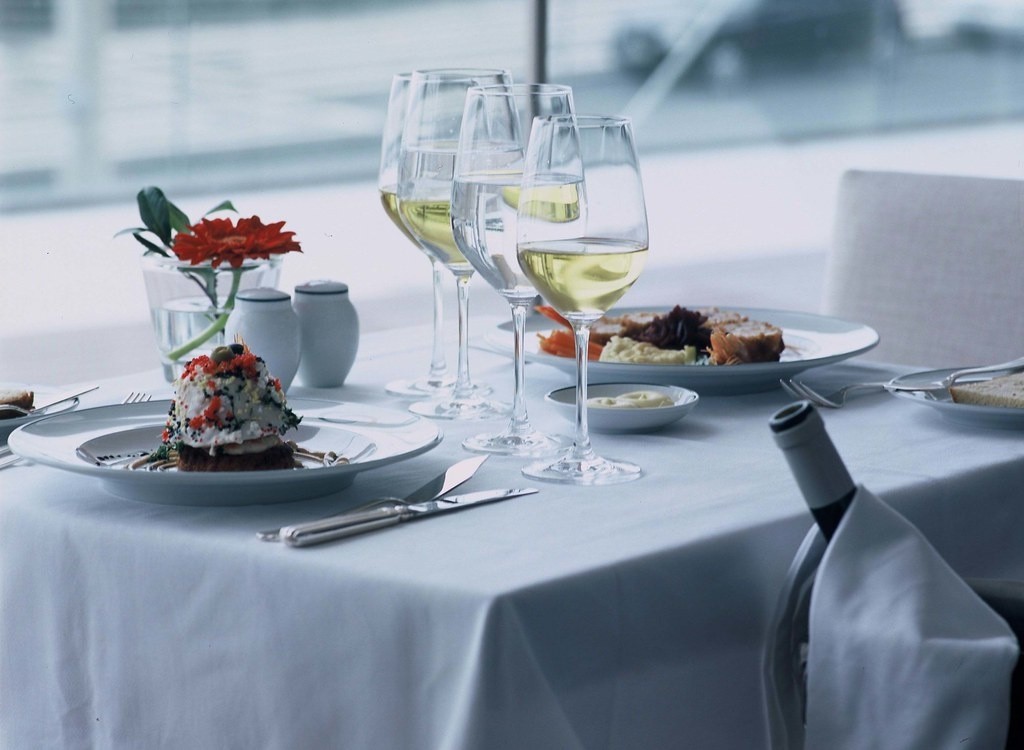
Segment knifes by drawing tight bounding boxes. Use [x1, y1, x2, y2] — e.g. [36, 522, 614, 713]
[279, 488, 541, 548]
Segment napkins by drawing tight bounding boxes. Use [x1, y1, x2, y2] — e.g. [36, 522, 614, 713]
[761, 481, 1017, 750]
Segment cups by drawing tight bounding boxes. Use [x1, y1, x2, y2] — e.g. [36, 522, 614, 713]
[140, 244, 271, 384]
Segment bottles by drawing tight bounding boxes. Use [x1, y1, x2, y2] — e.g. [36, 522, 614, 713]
[293, 279, 359, 389]
[771, 400, 859, 546]
[225, 287, 302, 397]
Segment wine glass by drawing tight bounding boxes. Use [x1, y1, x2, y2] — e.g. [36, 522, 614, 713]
[396, 68, 527, 419]
[377, 74, 492, 399]
[450, 82, 591, 459]
[518, 111, 650, 487]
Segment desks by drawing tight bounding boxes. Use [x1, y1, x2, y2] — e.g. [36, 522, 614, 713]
[0, 308, 1024, 750]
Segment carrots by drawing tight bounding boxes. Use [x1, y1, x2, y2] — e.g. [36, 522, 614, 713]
[534, 305, 604, 360]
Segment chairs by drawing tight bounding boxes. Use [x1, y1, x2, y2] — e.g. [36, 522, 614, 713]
[822, 168, 1024, 383]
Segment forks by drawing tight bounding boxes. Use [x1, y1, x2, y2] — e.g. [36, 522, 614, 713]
[778, 379, 890, 409]
[0, 392, 152, 470]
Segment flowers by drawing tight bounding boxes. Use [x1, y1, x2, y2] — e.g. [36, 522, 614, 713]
[116, 187, 303, 363]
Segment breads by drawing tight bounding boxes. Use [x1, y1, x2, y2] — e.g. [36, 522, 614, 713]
[949, 370, 1024, 411]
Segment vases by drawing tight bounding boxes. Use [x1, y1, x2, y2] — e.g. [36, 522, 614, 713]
[142, 257, 281, 381]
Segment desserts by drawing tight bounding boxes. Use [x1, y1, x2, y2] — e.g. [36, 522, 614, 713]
[596, 304, 785, 366]
[0, 386, 34, 420]
[153, 333, 303, 470]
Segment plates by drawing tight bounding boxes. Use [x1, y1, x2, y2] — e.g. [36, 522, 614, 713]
[0, 381, 80, 442]
[7, 399, 445, 508]
[462, 302, 881, 397]
[888, 366, 1024, 430]
[542, 382, 700, 435]
